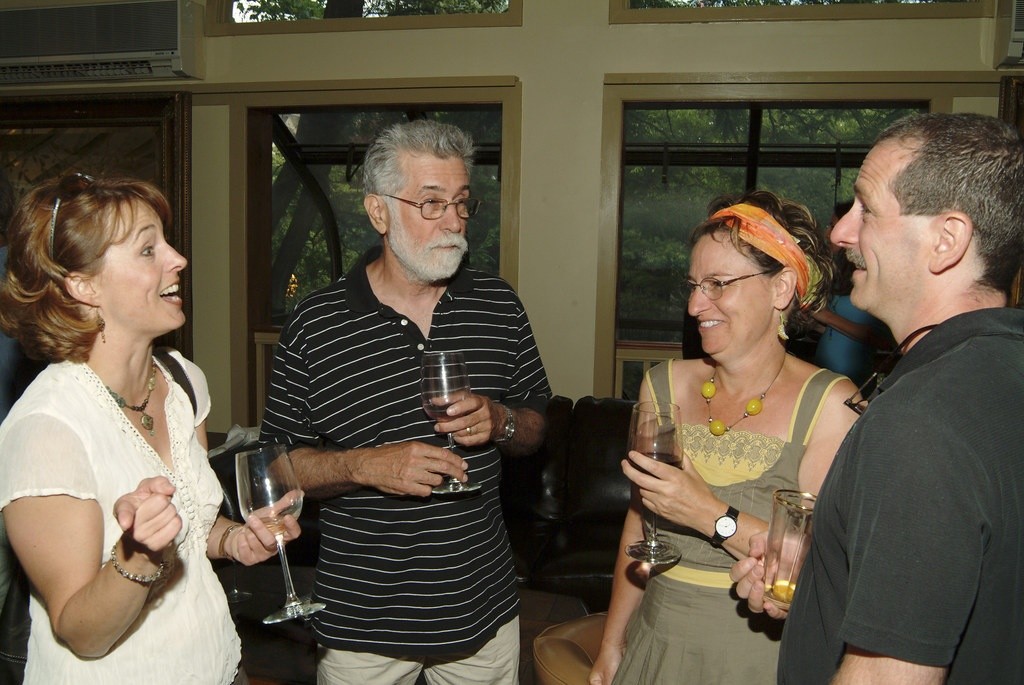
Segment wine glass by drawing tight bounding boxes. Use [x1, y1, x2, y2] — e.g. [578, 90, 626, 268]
[419, 351, 483, 495]
[623, 400, 684, 566]
[234, 443, 327, 624]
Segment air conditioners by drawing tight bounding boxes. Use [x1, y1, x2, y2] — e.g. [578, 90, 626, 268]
[0, 2, 206, 83]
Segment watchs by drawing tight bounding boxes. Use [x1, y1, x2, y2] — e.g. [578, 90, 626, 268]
[710, 506, 740, 548]
[495, 405, 516, 444]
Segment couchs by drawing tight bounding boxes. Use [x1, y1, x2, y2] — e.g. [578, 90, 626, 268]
[209, 393, 643, 603]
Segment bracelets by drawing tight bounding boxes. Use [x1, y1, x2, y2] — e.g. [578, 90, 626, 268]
[110, 541, 164, 583]
[218, 524, 243, 559]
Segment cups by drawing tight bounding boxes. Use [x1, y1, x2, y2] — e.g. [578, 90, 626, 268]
[759, 489, 818, 611]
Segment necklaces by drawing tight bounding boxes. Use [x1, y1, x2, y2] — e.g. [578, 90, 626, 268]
[700, 360, 786, 436]
[105, 368, 156, 436]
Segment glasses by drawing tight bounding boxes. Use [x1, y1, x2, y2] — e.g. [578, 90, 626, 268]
[845, 323, 941, 415]
[384, 193, 481, 220]
[49, 168, 103, 261]
[679, 268, 778, 300]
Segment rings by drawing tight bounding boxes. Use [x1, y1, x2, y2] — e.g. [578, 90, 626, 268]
[467, 427, 471, 435]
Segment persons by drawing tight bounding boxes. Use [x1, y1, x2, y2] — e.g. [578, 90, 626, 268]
[806, 201, 876, 399]
[729, 112, 1024, 685]
[0, 172, 306, 685]
[589, 190, 868, 685]
[255, 120, 552, 685]
